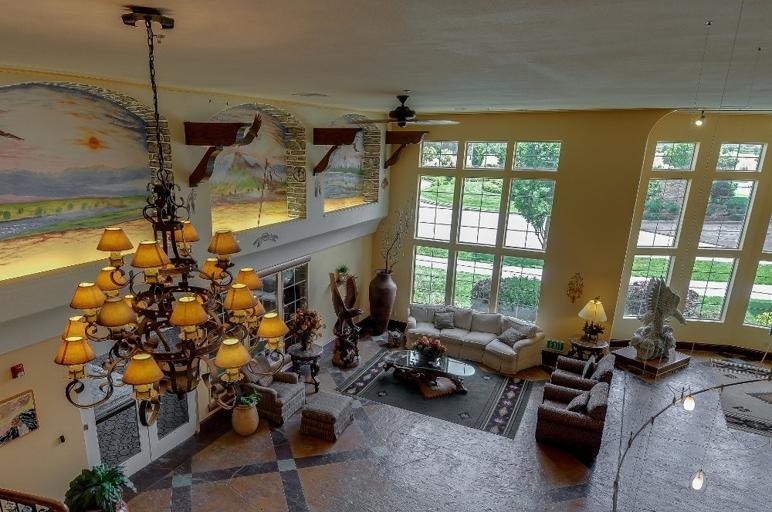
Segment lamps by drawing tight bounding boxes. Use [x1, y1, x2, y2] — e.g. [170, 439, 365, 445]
[577, 297, 608, 343]
[54, 3, 290, 427]
[688, 385, 723, 492]
[667, 383, 695, 411]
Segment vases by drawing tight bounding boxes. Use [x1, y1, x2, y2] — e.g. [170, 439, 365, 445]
[429, 357, 440, 367]
[298, 333, 313, 350]
[369, 267, 397, 335]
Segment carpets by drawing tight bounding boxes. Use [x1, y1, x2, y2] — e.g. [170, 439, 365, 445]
[333, 346, 533, 440]
[711, 358, 772, 437]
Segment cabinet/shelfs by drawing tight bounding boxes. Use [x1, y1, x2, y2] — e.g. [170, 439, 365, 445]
[611, 346, 691, 379]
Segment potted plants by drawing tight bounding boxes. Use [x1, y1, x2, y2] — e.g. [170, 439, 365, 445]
[232, 390, 263, 436]
[337, 264, 347, 282]
[64, 463, 137, 512]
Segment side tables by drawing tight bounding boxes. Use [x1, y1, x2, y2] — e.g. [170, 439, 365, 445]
[541, 349, 562, 373]
[569, 335, 608, 361]
[287, 343, 323, 393]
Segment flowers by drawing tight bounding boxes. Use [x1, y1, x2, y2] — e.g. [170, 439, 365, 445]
[378, 196, 413, 272]
[289, 305, 324, 339]
[415, 336, 447, 356]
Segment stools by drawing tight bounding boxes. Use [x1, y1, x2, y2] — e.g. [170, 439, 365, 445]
[300, 392, 353, 441]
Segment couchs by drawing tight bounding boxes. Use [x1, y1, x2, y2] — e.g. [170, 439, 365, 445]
[405, 304, 545, 374]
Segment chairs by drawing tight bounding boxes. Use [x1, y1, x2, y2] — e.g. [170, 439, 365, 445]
[550, 354, 616, 393]
[240, 348, 306, 424]
[535, 382, 610, 457]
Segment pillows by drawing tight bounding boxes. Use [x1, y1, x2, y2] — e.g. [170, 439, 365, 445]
[565, 390, 589, 411]
[495, 326, 526, 346]
[583, 355, 595, 379]
[435, 312, 455, 329]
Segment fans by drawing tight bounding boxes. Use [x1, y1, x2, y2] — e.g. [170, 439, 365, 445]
[351, 88, 460, 127]
